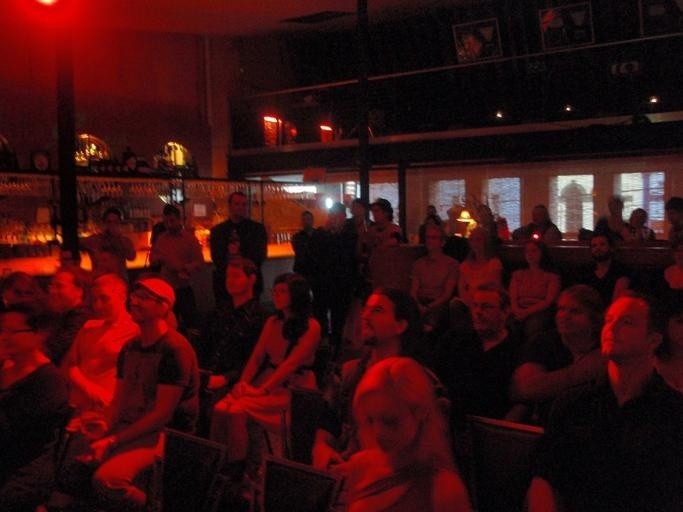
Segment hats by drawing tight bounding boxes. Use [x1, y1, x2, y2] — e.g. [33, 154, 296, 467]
[132, 277, 177, 306]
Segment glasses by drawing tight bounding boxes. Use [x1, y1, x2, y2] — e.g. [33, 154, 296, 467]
[133, 290, 162, 304]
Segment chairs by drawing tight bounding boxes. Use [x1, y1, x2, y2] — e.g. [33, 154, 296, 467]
[52, 348, 345, 512]
[463, 408, 548, 512]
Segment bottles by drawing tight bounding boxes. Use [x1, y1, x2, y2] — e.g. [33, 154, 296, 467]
[51, 426, 76, 460]
[87, 143, 150, 175]
[272, 231, 290, 244]
[99, 198, 150, 219]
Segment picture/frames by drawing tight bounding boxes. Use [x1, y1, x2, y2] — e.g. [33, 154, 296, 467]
[533, 2, 597, 54]
[635, 0, 682, 39]
[447, 13, 504, 63]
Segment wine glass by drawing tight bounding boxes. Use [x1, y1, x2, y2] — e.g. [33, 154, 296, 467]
[0, 175, 316, 200]
